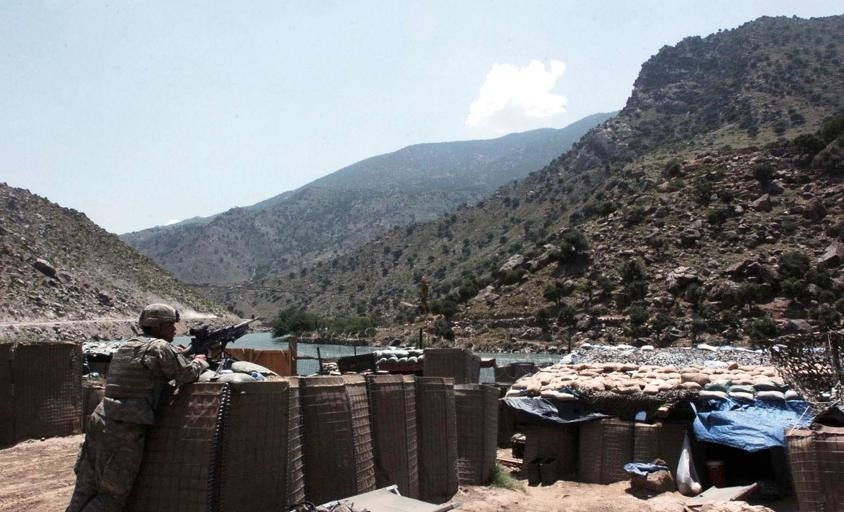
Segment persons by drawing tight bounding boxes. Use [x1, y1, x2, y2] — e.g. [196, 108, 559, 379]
[63, 303, 210, 512]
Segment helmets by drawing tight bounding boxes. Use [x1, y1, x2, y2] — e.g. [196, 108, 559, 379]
[139, 303, 180, 329]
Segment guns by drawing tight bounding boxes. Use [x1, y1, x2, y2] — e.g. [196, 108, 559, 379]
[183, 316, 259, 361]
